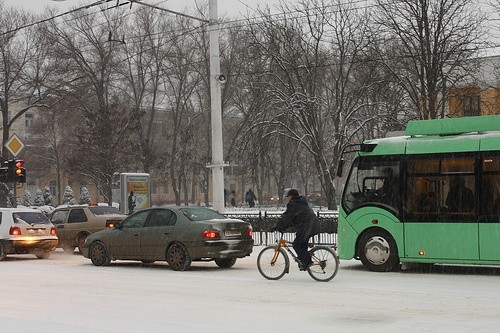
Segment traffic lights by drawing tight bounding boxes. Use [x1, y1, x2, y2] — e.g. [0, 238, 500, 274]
[15, 160, 25, 183]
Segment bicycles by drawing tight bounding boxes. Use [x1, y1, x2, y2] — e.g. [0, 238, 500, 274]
[257, 226, 340, 282]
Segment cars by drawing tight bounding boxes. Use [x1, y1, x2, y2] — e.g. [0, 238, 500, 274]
[17, 205, 55, 215]
[50, 205, 131, 251]
[82, 206, 254, 270]
[0, 208, 58, 260]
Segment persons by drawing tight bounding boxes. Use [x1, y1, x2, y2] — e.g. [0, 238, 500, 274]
[128, 190, 137, 217]
[228, 188, 259, 209]
[272, 189, 321, 269]
[366, 167, 500, 221]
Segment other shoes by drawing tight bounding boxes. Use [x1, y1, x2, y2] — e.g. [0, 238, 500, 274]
[299, 260, 314, 271]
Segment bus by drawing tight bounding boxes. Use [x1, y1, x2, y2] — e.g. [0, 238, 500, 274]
[336, 114, 500, 270]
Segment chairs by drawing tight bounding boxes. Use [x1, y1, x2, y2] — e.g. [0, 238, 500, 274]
[416, 192, 437, 211]
[151, 215, 165, 225]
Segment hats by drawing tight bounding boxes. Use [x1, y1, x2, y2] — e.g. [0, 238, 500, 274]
[285, 189, 300, 197]
